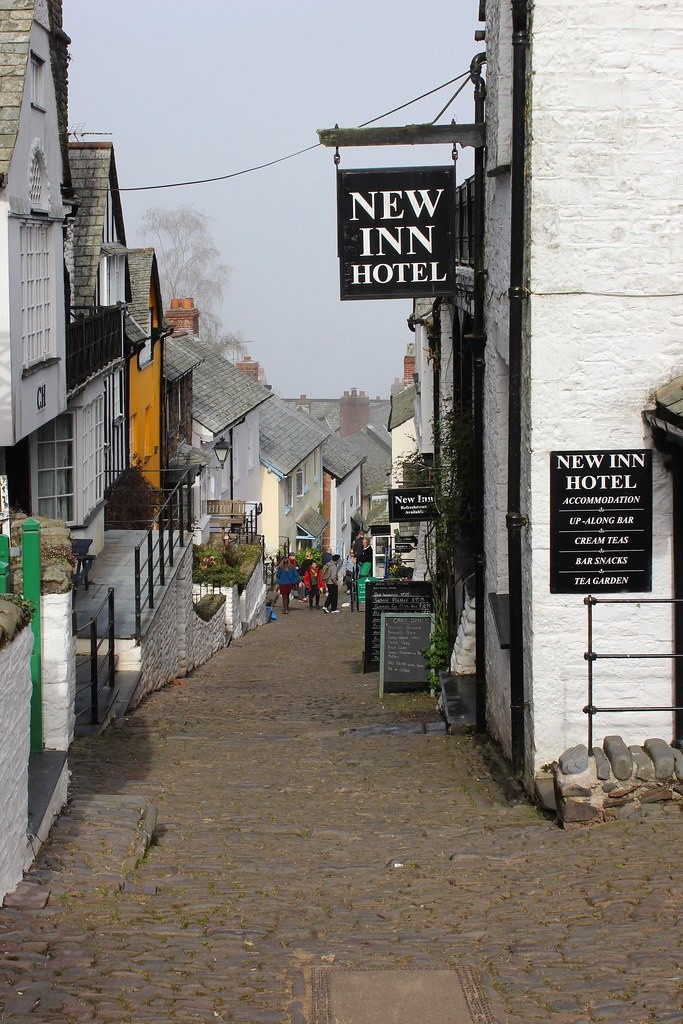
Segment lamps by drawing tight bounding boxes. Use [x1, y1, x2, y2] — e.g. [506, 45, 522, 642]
[211, 436, 233, 469]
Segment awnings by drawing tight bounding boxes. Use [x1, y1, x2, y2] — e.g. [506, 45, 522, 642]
[295, 508, 328, 539]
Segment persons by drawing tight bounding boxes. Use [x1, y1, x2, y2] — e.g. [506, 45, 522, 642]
[276, 556, 302, 614]
[288, 530, 372, 614]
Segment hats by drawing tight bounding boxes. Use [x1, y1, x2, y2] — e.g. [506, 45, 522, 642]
[288, 552, 295, 556]
[331, 555, 340, 561]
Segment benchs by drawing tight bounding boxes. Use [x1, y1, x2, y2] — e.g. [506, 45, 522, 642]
[72, 555, 95, 609]
[207, 500, 247, 546]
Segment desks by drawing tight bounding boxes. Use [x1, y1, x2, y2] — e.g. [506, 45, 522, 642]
[72, 539, 93, 609]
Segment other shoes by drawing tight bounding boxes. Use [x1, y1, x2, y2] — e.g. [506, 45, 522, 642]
[298, 600, 305, 602]
[282, 610, 289, 613]
[346, 590, 351, 595]
[303, 598, 308, 601]
[316, 605, 321, 610]
[310, 606, 314, 611]
[325, 592, 329, 597]
[331, 609, 340, 613]
[322, 606, 330, 614]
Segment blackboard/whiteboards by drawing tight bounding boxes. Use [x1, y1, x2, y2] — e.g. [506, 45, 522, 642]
[378, 612, 436, 683]
[363, 581, 433, 666]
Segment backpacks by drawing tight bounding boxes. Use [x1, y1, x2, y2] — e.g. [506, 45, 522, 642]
[321, 562, 335, 582]
